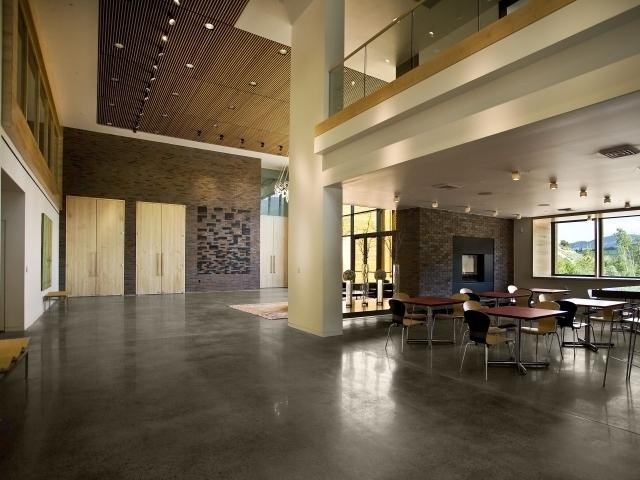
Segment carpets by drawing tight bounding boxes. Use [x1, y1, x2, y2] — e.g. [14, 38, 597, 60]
[227, 301, 288, 320]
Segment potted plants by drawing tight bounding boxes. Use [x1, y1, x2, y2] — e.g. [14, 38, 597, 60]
[343, 269, 355, 307]
[374, 269, 385, 304]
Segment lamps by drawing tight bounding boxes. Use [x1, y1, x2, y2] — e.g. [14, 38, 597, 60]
[603, 196, 611, 203]
[432, 200, 440, 208]
[550, 182, 558, 190]
[131, 0, 182, 134]
[464, 206, 470, 213]
[580, 189, 587, 197]
[274, 149, 291, 203]
[516, 214, 521, 220]
[393, 195, 400, 203]
[511, 171, 520, 180]
[492, 211, 497, 216]
[624, 202, 631, 209]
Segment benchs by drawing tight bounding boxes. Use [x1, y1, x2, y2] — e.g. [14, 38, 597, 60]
[1, 333, 35, 404]
[45, 290, 70, 312]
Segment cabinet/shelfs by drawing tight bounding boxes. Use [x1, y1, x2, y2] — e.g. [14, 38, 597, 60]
[66, 195, 125, 296]
[133, 201, 186, 296]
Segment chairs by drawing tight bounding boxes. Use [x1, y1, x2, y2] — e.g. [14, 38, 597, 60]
[382, 283, 639, 387]
